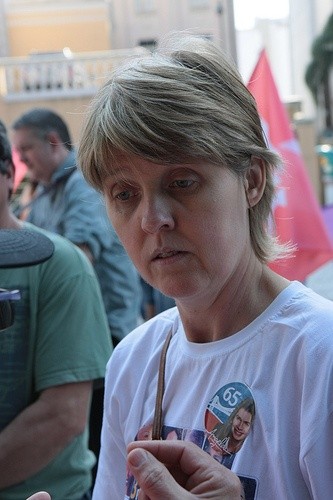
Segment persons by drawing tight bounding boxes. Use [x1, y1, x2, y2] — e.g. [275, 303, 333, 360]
[123, 433, 247, 500]
[140, 284, 176, 317]
[13, 109, 143, 496]
[0, 121, 114, 500]
[26, 51, 333, 500]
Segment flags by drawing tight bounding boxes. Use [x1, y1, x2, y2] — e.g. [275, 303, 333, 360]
[246, 52, 332, 284]
